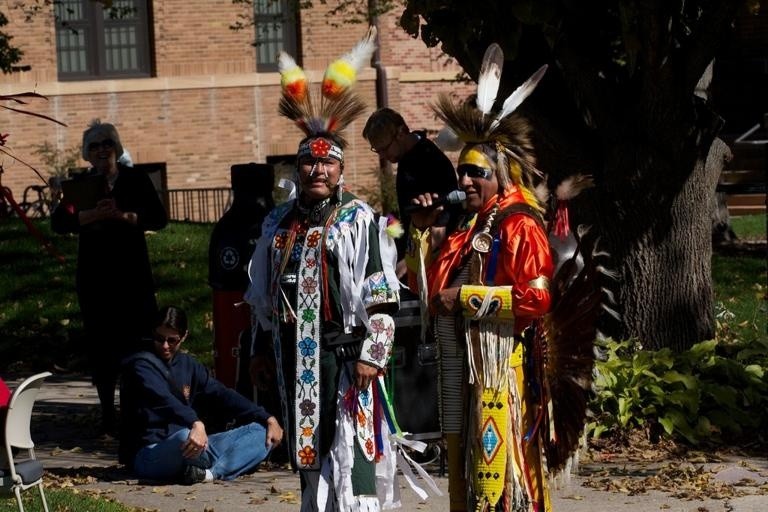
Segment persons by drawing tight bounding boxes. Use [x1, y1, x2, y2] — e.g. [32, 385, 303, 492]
[118, 306, 284, 485]
[52, 117, 170, 416]
[361, 109, 463, 285]
[405, 144, 559, 511]
[242, 135, 445, 510]
[208, 161, 289, 472]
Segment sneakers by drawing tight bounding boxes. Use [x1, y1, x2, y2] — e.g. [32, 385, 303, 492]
[188, 465, 206, 484]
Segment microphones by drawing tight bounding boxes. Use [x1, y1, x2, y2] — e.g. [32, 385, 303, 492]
[404, 189, 467, 214]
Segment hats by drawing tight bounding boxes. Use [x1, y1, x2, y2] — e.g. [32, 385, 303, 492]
[429, 90, 547, 205]
[279, 86, 370, 162]
[83, 123, 122, 160]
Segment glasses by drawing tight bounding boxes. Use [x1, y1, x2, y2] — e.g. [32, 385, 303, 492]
[457, 165, 491, 180]
[371, 137, 395, 154]
[87, 140, 114, 152]
[154, 332, 181, 346]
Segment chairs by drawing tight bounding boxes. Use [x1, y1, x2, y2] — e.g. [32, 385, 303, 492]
[0, 371, 58, 512]
